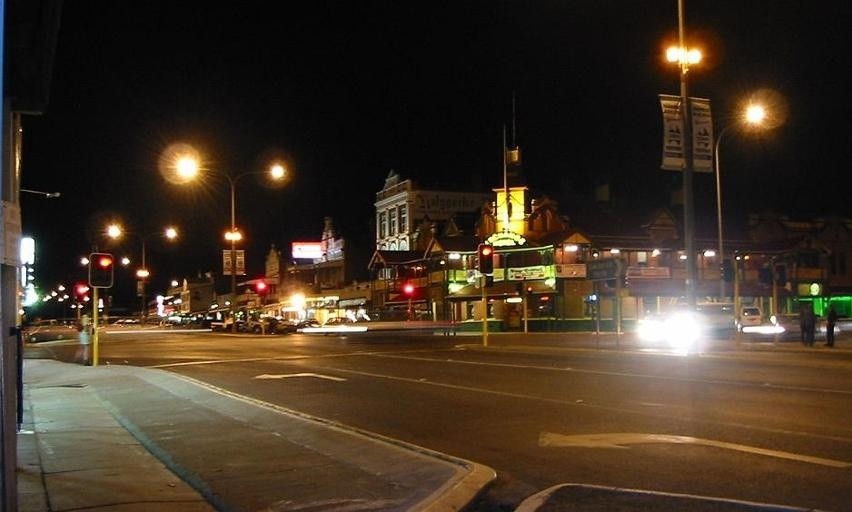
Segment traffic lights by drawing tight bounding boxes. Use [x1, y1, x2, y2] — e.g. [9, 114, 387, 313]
[478, 243, 494, 274]
[88, 253, 115, 288]
[72, 282, 90, 303]
[258, 281, 267, 290]
[405, 285, 415, 293]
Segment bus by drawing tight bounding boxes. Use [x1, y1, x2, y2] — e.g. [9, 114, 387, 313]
[672, 302, 747, 332]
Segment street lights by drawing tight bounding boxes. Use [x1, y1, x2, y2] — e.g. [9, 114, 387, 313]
[177, 156, 286, 330]
[713, 108, 763, 296]
[108, 226, 176, 315]
[665, 46, 704, 357]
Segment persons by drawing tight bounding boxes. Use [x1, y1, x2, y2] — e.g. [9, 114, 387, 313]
[74, 307, 92, 365]
[823, 304, 838, 347]
[799, 304, 817, 346]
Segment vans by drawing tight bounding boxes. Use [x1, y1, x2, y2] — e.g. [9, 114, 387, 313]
[737, 307, 764, 329]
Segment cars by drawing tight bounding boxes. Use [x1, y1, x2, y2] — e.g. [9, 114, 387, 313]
[245, 313, 318, 334]
[21, 319, 98, 342]
